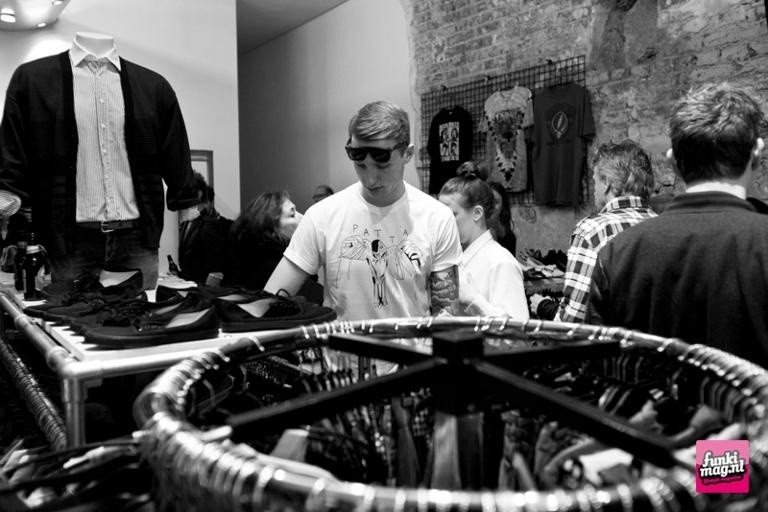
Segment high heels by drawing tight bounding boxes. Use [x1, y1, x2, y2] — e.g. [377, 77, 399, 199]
[14, 245, 52, 300]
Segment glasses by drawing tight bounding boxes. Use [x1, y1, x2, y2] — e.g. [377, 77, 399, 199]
[345, 137, 408, 163]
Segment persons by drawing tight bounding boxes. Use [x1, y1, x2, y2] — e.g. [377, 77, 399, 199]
[179, 99, 530, 378]
[529, 81, 768, 371]
[0, 31, 207, 290]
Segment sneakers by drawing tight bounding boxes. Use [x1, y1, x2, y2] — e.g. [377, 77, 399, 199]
[221, 287, 337, 334]
[518, 246, 568, 281]
[24, 269, 236, 349]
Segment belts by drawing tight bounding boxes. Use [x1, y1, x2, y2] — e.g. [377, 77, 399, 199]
[69, 221, 135, 235]
